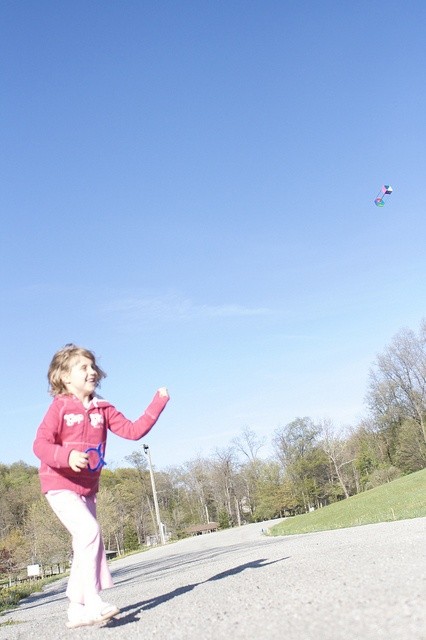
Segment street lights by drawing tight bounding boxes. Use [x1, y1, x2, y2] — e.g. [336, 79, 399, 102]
[143, 444, 165, 544]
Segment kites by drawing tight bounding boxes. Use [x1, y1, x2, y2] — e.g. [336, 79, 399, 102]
[374, 185, 393, 207]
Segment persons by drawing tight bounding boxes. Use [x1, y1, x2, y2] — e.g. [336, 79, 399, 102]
[33, 344, 170, 629]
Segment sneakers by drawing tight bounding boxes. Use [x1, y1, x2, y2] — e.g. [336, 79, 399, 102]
[67, 610, 95, 628]
[86, 603, 117, 622]
